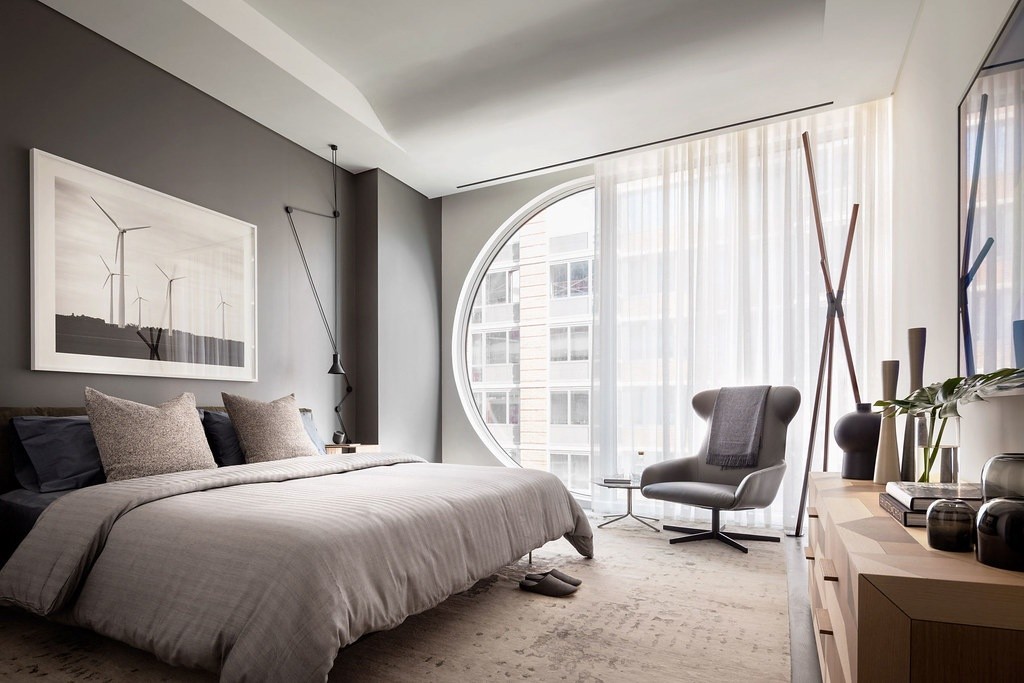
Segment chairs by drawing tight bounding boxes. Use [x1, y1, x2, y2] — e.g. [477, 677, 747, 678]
[640, 385, 801, 553]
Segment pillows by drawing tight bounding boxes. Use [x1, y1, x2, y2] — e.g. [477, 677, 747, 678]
[85, 384, 218, 482]
[203, 410, 246, 469]
[12, 416, 105, 494]
[222, 391, 320, 463]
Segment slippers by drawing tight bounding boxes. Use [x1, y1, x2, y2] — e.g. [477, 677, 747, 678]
[519, 574, 578, 596]
[525, 569, 582, 586]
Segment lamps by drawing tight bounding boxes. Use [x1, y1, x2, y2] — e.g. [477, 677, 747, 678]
[327, 353, 353, 445]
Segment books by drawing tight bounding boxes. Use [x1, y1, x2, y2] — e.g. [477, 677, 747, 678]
[604, 478, 631, 484]
[886, 480, 984, 511]
[879, 493, 926, 527]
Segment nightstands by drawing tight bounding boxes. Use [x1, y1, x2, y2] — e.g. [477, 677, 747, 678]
[325, 444, 380, 455]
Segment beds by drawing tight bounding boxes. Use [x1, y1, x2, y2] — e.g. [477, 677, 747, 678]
[0, 406, 593, 683]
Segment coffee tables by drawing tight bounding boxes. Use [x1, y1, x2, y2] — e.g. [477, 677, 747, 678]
[591, 477, 661, 533]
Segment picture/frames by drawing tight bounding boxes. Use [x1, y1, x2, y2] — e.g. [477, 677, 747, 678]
[30, 149, 258, 381]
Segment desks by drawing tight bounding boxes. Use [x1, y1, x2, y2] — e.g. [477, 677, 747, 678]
[803, 471, 1024, 683]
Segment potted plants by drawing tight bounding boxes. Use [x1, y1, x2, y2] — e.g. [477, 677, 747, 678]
[872, 368, 1024, 482]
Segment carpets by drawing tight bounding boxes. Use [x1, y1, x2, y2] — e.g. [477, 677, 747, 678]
[326, 518, 791, 683]
